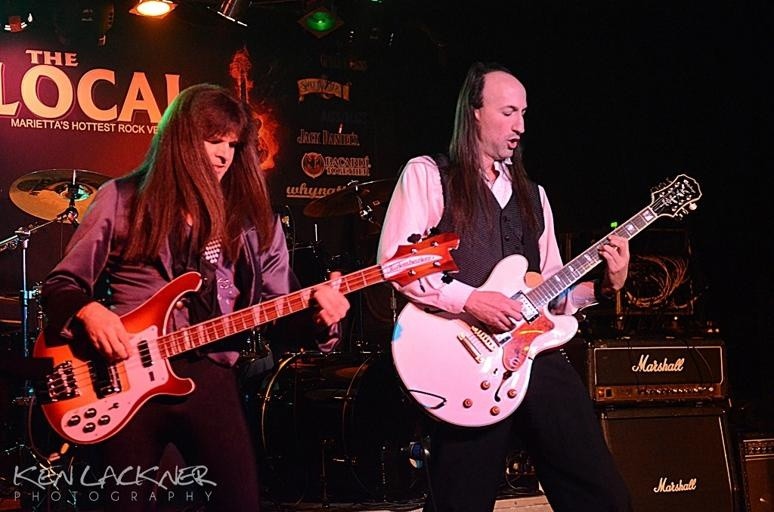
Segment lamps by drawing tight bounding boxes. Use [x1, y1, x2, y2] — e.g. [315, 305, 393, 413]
[297, 1, 350, 40]
[208, 0, 259, 26]
[2, 0, 34, 33]
[127, 0, 178, 21]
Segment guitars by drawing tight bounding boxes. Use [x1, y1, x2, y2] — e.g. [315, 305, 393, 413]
[31, 232, 460, 445]
[393, 173, 703, 428]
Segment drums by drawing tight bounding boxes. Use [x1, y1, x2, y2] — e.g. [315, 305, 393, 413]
[0, 297, 36, 499]
[262, 344, 424, 502]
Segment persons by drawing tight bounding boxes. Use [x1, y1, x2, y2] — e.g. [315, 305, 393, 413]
[42, 85, 351, 512]
[376, 62, 630, 512]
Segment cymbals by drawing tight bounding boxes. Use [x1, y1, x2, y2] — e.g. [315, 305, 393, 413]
[8, 166, 113, 226]
[304, 179, 394, 218]
